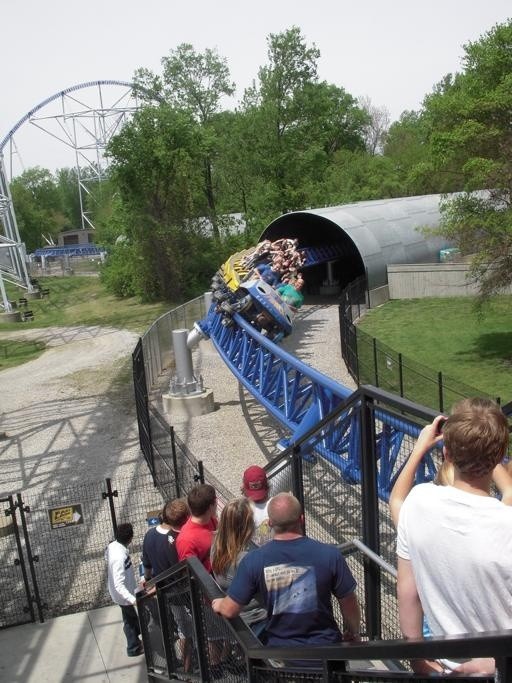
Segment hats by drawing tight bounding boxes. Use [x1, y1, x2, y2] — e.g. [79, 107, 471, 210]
[243, 465, 267, 500]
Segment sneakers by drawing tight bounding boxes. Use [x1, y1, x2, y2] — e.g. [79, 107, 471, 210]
[211, 655, 241, 680]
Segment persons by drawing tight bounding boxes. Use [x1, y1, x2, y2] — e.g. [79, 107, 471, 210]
[394, 395, 511, 676]
[241, 234, 309, 311]
[385, 414, 511, 530]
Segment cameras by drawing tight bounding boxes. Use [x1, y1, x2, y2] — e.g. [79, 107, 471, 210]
[438, 419, 446, 435]
